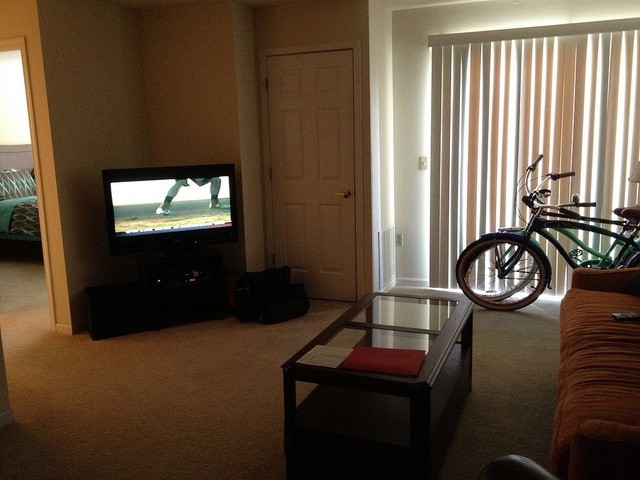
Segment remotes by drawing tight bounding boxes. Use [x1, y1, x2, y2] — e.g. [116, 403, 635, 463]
[612, 312, 640, 320]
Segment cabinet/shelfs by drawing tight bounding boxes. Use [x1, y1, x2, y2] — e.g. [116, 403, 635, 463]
[83, 268, 238, 341]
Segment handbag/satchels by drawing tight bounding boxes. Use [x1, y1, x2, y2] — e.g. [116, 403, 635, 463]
[217, 265, 311, 326]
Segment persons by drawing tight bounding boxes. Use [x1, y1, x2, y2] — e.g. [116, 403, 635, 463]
[155, 176, 222, 215]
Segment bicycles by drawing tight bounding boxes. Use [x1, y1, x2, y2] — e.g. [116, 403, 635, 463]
[456, 154, 640, 302]
[456, 172, 640, 311]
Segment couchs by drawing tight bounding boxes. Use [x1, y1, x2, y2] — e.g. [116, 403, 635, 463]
[548, 267, 640, 480]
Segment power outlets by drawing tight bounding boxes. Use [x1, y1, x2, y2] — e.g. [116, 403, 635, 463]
[395, 233, 403, 245]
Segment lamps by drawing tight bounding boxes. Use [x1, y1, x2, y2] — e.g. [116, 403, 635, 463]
[628, 161, 640, 184]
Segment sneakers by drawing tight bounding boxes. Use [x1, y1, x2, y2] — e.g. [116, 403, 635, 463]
[209, 204, 230, 209]
[156, 207, 171, 215]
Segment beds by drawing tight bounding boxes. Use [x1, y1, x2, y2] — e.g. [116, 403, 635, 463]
[0, 144, 42, 242]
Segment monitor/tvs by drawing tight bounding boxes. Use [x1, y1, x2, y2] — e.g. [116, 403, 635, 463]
[102, 163, 239, 256]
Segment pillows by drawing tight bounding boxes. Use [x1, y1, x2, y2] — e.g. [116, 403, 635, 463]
[0, 169, 38, 201]
